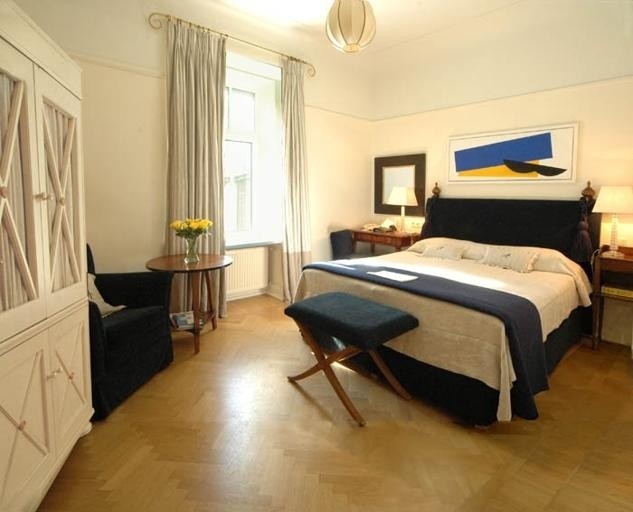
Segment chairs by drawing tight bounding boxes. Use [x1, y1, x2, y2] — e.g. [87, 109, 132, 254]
[332, 229, 365, 260]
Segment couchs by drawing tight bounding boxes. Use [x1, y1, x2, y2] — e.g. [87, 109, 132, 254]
[74, 244, 174, 417]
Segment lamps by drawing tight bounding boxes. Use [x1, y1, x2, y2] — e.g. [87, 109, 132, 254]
[324, 1, 377, 55]
[592, 185, 633, 259]
[384, 186, 419, 233]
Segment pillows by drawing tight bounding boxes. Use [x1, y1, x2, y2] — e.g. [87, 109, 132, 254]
[480, 243, 536, 271]
[423, 238, 467, 259]
[520, 220, 572, 248]
[448, 214, 487, 234]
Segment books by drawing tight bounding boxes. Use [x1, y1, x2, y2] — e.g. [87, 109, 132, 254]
[601, 281, 633, 299]
[169, 311, 194, 331]
[172, 313, 204, 328]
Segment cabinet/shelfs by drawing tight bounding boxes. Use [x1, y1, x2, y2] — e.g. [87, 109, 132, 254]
[0, 0, 95, 512]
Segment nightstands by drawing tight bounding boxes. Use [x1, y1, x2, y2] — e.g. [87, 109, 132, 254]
[593, 245, 633, 350]
[353, 230, 422, 251]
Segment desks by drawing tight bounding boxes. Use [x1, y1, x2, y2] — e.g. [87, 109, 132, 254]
[146, 254, 233, 353]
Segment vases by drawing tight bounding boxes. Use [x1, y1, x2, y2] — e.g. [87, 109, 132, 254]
[184, 237, 202, 264]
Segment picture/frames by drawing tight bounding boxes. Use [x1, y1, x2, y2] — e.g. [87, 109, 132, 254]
[447, 123, 576, 182]
[373, 153, 426, 218]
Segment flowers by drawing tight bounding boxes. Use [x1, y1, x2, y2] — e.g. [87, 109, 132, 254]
[170, 218, 213, 253]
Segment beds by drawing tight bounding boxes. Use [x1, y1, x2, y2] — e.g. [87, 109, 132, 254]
[303, 182, 596, 425]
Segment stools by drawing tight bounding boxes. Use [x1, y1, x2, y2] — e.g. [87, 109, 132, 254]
[286, 292, 418, 427]
